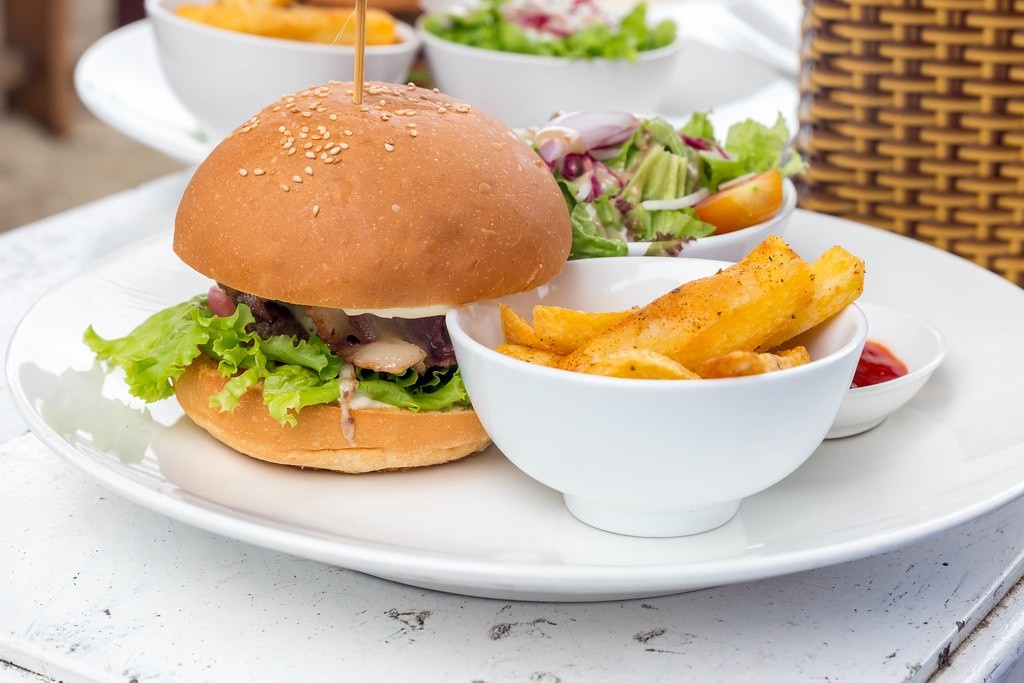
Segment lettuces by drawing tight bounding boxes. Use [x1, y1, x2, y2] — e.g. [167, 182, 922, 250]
[421, 0, 677, 63]
[554, 112, 789, 258]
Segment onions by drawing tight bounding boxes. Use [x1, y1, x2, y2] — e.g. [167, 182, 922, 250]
[518, 108, 730, 211]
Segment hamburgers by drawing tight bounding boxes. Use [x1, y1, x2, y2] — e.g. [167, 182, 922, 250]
[81, 79, 571, 473]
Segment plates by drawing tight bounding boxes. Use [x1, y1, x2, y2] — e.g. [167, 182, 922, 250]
[5, 210, 1024, 601]
[74, 19, 800, 160]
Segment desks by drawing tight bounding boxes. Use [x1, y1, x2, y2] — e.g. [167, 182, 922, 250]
[1, 167, 1024, 683]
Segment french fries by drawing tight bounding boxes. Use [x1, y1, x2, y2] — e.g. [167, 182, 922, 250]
[176, 0, 399, 46]
[490, 236, 866, 380]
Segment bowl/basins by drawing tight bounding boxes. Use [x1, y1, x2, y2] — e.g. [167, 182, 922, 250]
[445, 257, 866, 538]
[146, 0, 420, 133]
[822, 302, 944, 439]
[418, 16, 680, 132]
[624, 174, 796, 264]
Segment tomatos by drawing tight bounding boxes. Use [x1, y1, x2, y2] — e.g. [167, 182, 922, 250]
[692, 170, 783, 234]
